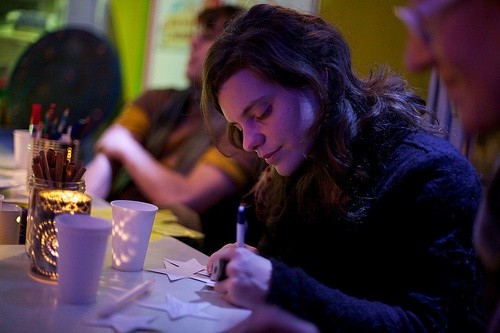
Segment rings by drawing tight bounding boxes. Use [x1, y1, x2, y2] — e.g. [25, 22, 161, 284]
[212, 257, 230, 281]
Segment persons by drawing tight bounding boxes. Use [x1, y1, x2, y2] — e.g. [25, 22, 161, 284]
[201, 3, 500, 333]
[391, 0, 500, 272]
[81, 5, 267, 256]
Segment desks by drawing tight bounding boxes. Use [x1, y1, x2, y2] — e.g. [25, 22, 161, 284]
[0, 169, 319, 333]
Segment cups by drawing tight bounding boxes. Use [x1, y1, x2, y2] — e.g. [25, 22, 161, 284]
[110, 200, 159, 272]
[28, 190, 92, 285]
[13, 129, 31, 168]
[25, 173, 86, 256]
[0, 174, 29, 245]
[26, 135, 80, 190]
[54, 214, 112, 304]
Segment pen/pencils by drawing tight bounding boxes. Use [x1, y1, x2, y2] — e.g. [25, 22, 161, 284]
[99, 277, 156, 317]
[29, 104, 92, 145]
[237, 205, 245, 246]
[29, 148, 87, 183]
